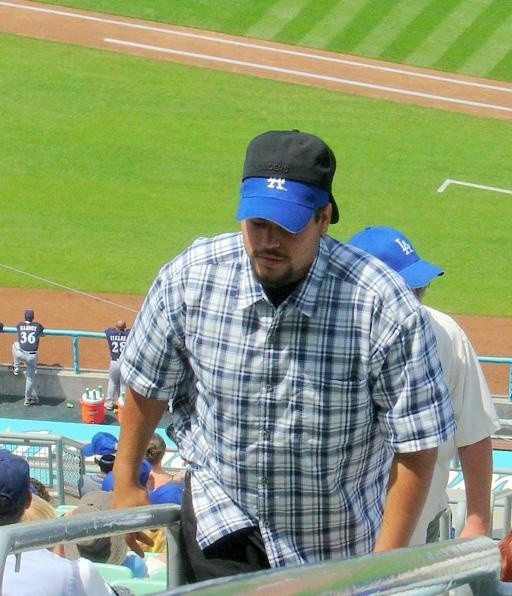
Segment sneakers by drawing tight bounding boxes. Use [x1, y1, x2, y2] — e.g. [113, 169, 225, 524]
[104, 403, 112, 410]
[24, 398, 35, 407]
[8, 366, 19, 376]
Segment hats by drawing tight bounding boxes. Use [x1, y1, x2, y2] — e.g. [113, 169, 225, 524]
[0, 447, 31, 515]
[139, 459, 152, 487]
[345, 223, 446, 289]
[25, 310, 34, 321]
[60, 489, 128, 566]
[234, 128, 339, 233]
[81, 432, 118, 457]
[97, 454, 115, 469]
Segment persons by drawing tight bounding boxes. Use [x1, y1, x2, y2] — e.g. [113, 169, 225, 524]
[77, 431, 118, 498]
[145, 432, 180, 488]
[63, 491, 149, 581]
[19, 493, 106, 595]
[102, 460, 184, 505]
[347, 224, 501, 546]
[112, 128, 458, 582]
[104, 320, 132, 409]
[0, 448, 78, 596]
[8, 309, 44, 406]
